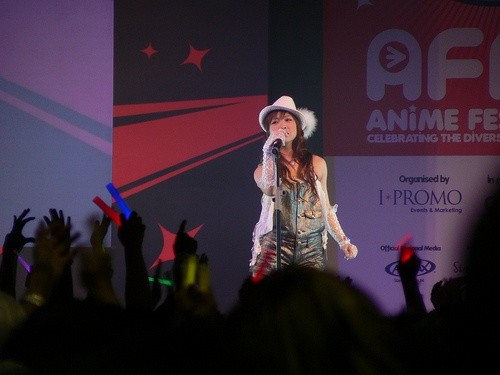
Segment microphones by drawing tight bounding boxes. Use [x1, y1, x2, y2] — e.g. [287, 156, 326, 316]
[272, 138, 282, 154]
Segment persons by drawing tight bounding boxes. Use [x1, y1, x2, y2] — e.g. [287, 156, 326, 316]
[0, 192, 500, 375]
[250, 95, 357, 282]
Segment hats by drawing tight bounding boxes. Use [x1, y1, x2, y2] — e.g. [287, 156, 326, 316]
[258, 96, 318, 140]
